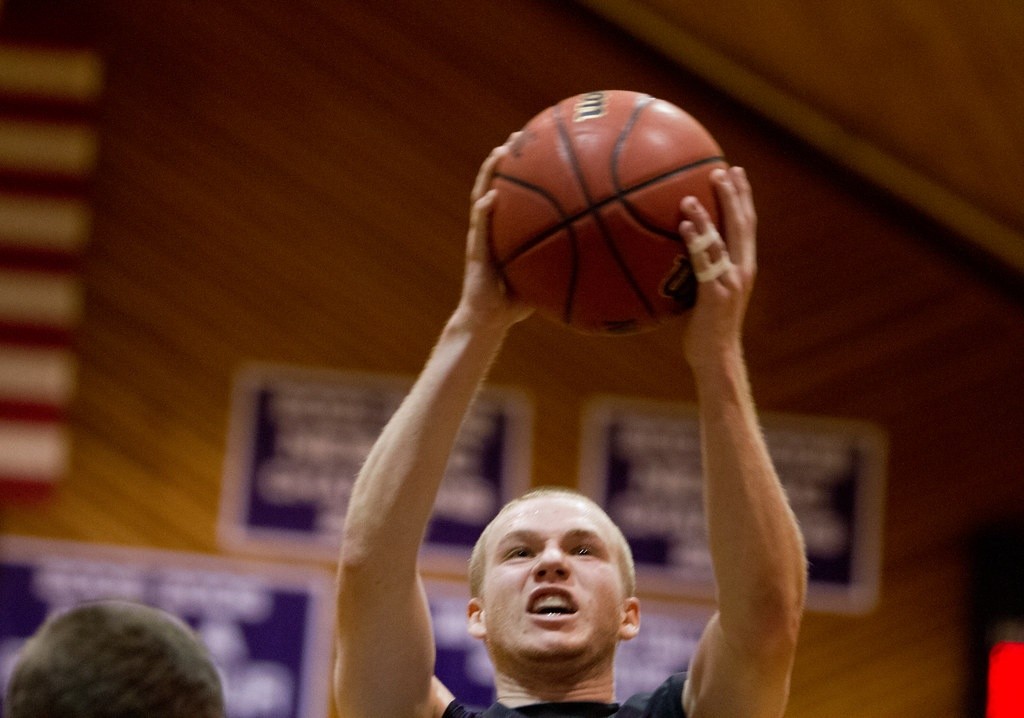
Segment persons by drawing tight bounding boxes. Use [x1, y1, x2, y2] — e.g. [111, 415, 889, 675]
[3, 601, 226, 718]
[335, 132, 807, 718]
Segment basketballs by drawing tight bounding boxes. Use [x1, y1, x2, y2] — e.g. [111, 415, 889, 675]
[487, 88, 729, 336]
